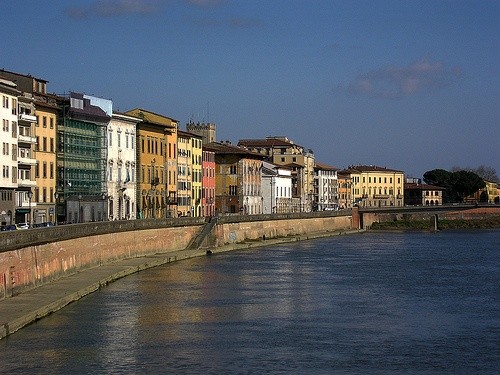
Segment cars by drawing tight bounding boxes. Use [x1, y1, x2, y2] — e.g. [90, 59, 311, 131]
[32, 222, 53, 229]
[18, 223, 30, 230]
[1, 225, 17, 231]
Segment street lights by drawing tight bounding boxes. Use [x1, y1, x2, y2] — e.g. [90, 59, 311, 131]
[101, 194, 106, 222]
[27, 190, 34, 229]
[54, 192, 58, 226]
[77, 192, 83, 223]
[123, 193, 340, 223]
